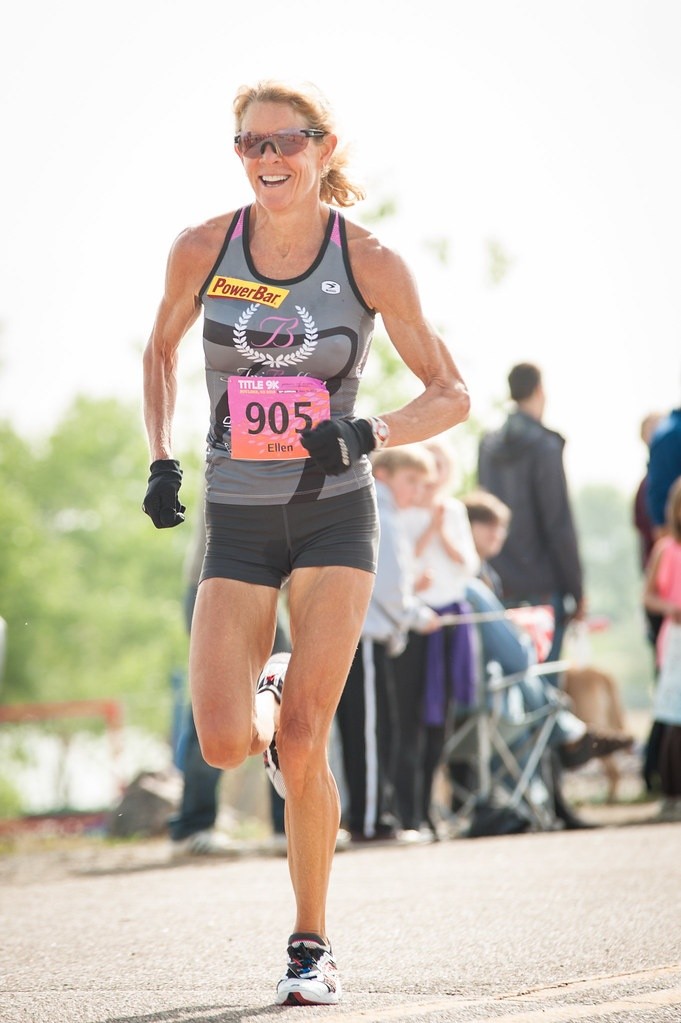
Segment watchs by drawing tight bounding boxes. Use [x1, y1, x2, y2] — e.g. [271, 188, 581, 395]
[369, 415, 389, 450]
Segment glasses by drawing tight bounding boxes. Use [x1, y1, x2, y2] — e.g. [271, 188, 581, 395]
[235, 128, 327, 157]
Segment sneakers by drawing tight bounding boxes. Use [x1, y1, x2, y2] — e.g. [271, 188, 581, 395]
[255, 648, 293, 799]
[274, 932, 342, 1007]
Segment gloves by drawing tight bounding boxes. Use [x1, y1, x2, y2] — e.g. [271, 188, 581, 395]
[301, 416, 377, 477]
[143, 458, 187, 528]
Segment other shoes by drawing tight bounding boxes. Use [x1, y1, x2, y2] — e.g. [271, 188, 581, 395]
[169, 794, 604, 855]
[558, 727, 634, 771]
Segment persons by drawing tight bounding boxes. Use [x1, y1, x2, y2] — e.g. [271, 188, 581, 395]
[141, 84, 470, 1004]
[167, 363, 681, 864]
